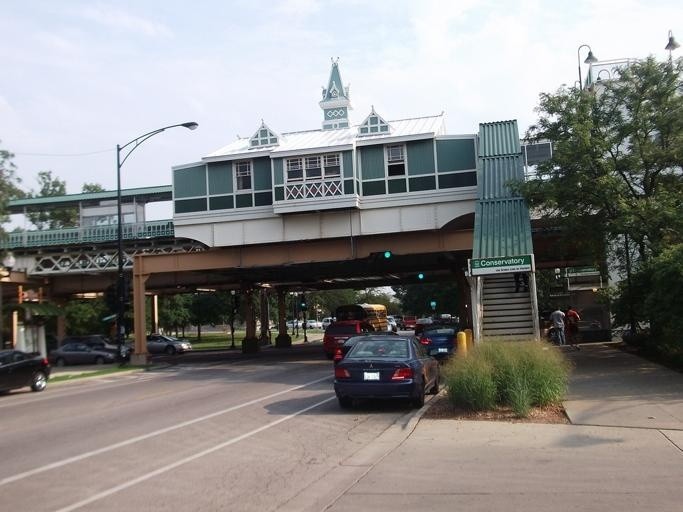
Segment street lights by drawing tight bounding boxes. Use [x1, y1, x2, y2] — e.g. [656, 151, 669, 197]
[117, 121, 199, 333]
[574, 45, 610, 96]
[666, 29, 679, 65]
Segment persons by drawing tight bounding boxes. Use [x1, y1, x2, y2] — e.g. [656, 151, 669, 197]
[549, 303, 581, 351]
[513, 272, 529, 293]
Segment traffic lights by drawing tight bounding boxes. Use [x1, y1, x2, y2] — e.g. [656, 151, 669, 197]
[428, 298, 439, 309]
[300, 298, 307, 311]
[370, 251, 392, 262]
[408, 273, 425, 282]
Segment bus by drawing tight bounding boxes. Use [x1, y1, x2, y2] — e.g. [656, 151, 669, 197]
[335, 304, 388, 331]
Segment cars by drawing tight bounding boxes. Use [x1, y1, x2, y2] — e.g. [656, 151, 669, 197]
[0, 333, 192, 393]
[548, 320, 603, 344]
[285, 315, 459, 409]
